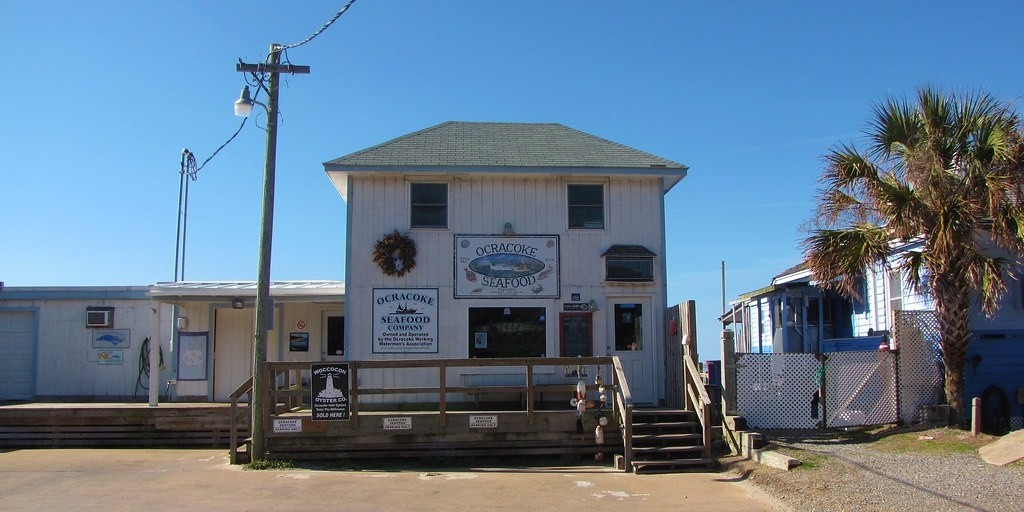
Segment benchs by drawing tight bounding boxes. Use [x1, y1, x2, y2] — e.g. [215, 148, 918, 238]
[467, 385, 586, 410]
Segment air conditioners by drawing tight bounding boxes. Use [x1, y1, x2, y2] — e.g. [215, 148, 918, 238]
[86, 310, 111, 326]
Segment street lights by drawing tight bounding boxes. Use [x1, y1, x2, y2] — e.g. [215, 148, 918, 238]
[233, 85, 279, 466]
